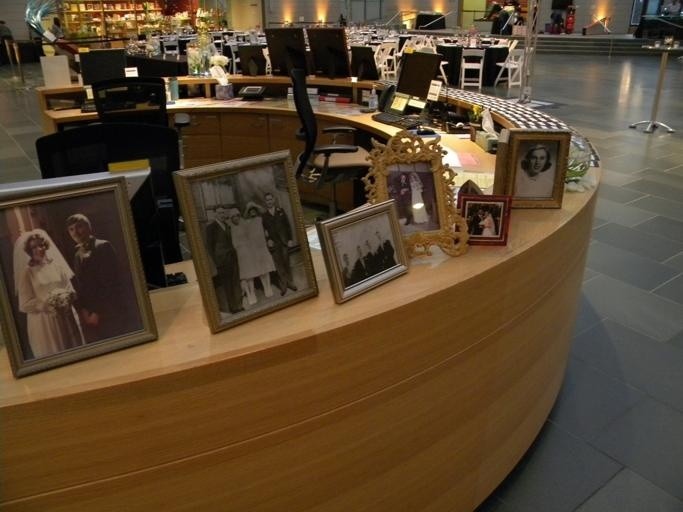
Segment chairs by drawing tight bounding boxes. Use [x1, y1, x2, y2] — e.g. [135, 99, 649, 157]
[286, 68, 375, 223]
[33, 75, 183, 266]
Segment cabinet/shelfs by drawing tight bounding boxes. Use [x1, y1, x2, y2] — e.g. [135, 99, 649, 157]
[58, 0, 200, 39]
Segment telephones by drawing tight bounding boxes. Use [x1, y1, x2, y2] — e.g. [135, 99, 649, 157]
[379, 85, 410, 115]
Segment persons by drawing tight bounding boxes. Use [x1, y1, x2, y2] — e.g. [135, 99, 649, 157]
[516, 144, 554, 199]
[10, 228, 81, 363]
[242, 199, 277, 299]
[0, 20, 14, 66]
[467, 209, 484, 235]
[261, 191, 298, 297]
[398, 172, 411, 226]
[339, 230, 394, 287]
[478, 209, 498, 236]
[61, 213, 138, 345]
[227, 206, 260, 307]
[407, 172, 431, 225]
[48, 17, 64, 40]
[204, 205, 245, 316]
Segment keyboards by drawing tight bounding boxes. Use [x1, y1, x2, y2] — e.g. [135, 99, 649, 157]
[81, 100, 137, 114]
[371, 112, 422, 130]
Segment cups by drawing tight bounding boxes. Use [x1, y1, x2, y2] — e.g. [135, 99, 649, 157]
[654, 41, 661, 47]
[674, 41, 680, 48]
[362, 90, 370, 107]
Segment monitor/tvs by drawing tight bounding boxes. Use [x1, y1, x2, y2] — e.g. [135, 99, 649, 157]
[395, 52, 442, 103]
[79, 47, 127, 86]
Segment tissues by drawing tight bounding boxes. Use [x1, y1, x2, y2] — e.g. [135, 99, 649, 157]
[208, 65, 234, 100]
[475, 108, 501, 153]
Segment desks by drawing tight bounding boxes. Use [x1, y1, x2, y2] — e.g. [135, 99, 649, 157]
[628, 41, 683, 134]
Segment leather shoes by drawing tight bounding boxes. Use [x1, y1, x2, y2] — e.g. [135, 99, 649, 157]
[247, 294, 258, 306]
[264, 288, 274, 297]
[280, 282, 298, 295]
[231, 307, 245, 314]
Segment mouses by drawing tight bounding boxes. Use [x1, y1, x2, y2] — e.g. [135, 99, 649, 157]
[417, 128, 435, 135]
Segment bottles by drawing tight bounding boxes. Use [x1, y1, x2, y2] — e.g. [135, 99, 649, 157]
[168, 77, 178, 101]
[306, 88, 319, 106]
[287, 87, 293, 100]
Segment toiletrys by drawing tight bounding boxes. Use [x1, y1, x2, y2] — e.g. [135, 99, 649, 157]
[369, 85, 379, 108]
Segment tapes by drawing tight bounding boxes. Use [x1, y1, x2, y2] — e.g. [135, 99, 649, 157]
[456, 122, 464, 127]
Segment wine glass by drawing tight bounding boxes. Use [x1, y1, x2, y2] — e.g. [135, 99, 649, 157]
[490, 39, 495, 46]
[453, 25, 482, 46]
